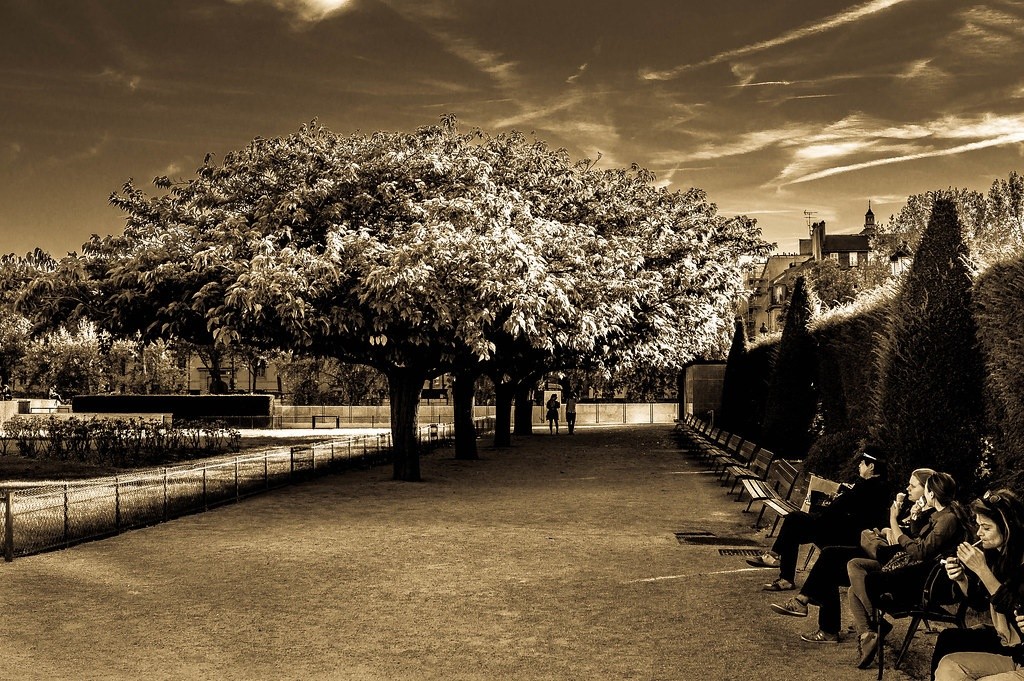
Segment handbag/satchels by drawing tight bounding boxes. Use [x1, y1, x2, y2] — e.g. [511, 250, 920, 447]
[807, 490, 836, 520]
[861, 528, 888, 560]
[880, 552, 924, 572]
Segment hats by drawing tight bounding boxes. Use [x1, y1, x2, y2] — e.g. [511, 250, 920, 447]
[860, 446, 886, 463]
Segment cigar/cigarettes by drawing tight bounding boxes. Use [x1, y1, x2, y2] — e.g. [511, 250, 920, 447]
[971, 539, 982, 548]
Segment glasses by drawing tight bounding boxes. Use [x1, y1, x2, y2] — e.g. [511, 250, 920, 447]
[982, 490, 1011, 511]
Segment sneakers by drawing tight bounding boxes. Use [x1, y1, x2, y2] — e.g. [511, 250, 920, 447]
[801, 629, 839, 644]
[770, 598, 808, 616]
[865, 619, 893, 639]
[763, 578, 795, 590]
[855, 631, 880, 668]
[745, 551, 781, 568]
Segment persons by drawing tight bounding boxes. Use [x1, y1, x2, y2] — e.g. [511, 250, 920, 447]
[931, 488, 1024, 681]
[847, 472, 975, 670]
[933, 610, 1024, 681]
[770, 469, 938, 643]
[3, 384, 12, 402]
[565, 391, 579, 435]
[49, 384, 69, 405]
[546, 394, 560, 436]
[746, 446, 889, 591]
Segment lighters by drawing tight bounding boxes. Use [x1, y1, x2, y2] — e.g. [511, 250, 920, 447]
[936, 553, 959, 567]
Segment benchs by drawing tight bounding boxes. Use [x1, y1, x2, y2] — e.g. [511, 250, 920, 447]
[871, 524, 985, 666]
[696, 431, 729, 458]
[677, 419, 702, 438]
[674, 416, 698, 434]
[686, 425, 714, 448]
[713, 440, 757, 480]
[732, 457, 800, 513]
[672, 412, 693, 431]
[688, 428, 721, 456]
[721, 448, 774, 495]
[681, 422, 707, 444]
[753, 472, 828, 537]
[706, 434, 742, 469]
